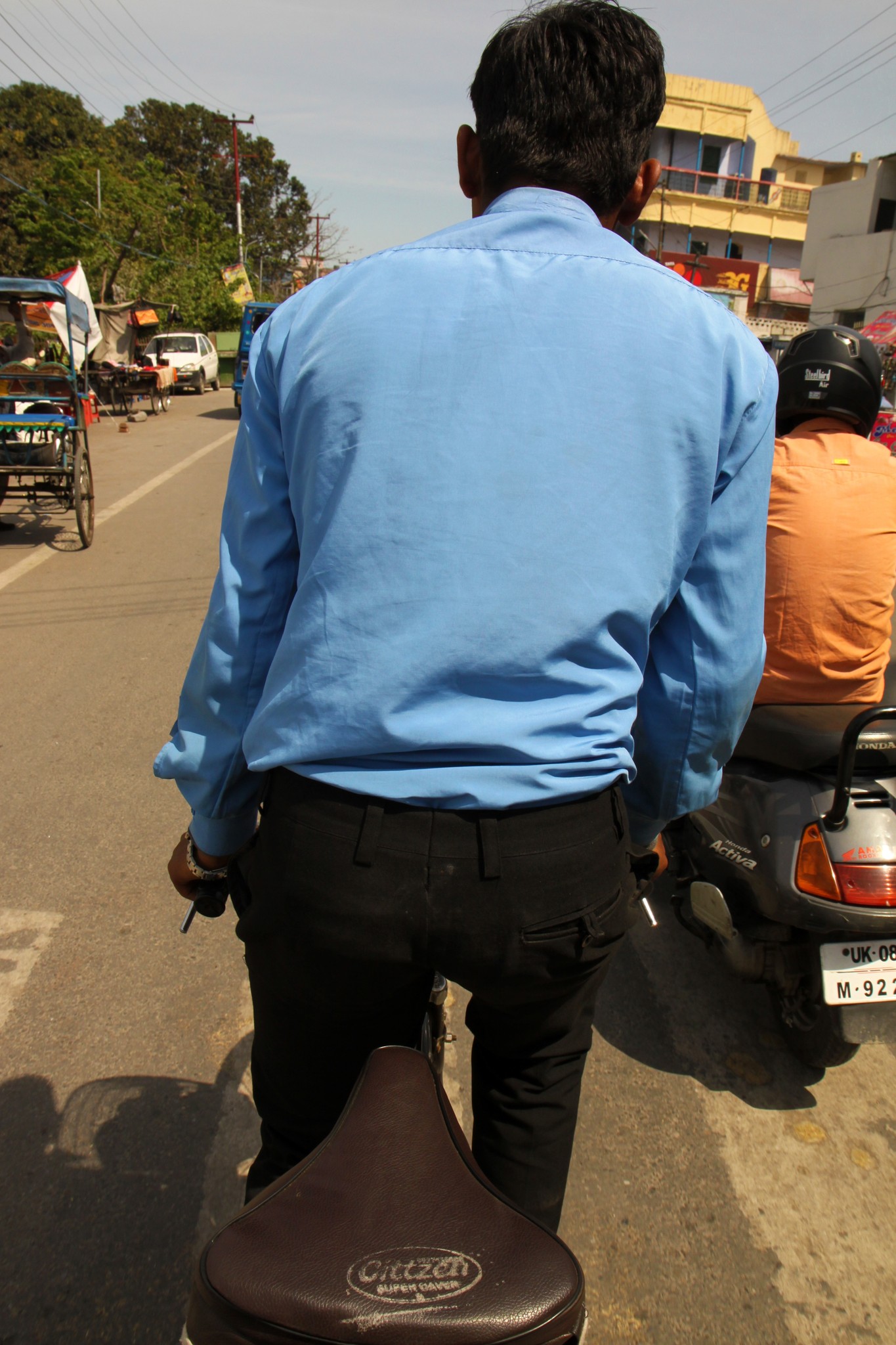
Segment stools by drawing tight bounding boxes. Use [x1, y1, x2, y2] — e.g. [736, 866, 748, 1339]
[82, 394, 101, 428]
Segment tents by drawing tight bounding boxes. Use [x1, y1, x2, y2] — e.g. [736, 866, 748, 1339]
[91, 296, 178, 371]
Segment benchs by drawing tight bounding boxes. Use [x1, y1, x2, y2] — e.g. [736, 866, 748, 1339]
[0, 361, 79, 429]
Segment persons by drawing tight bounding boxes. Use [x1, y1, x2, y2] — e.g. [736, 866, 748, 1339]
[157, 0, 779, 1234]
[653, 325, 896, 878]
[0, 295, 34, 440]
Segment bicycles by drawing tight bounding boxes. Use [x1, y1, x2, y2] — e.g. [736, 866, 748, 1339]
[178, 852, 659, 1345]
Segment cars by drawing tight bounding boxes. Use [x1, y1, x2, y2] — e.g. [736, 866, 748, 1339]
[141, 332, 221, 396]
[232, 301, 280, 421]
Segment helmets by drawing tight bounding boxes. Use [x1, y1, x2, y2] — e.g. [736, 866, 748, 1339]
[776, 325, 883, 439]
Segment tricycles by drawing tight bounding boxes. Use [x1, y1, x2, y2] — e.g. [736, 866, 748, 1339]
[0, 275, 96, 549]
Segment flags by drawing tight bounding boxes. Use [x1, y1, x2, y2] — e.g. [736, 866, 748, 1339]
[23, 264, 103, 371]
[220, 264, 255, 308]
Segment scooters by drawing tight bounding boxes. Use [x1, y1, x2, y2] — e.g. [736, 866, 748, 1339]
[652, 702, 896, 1069]
